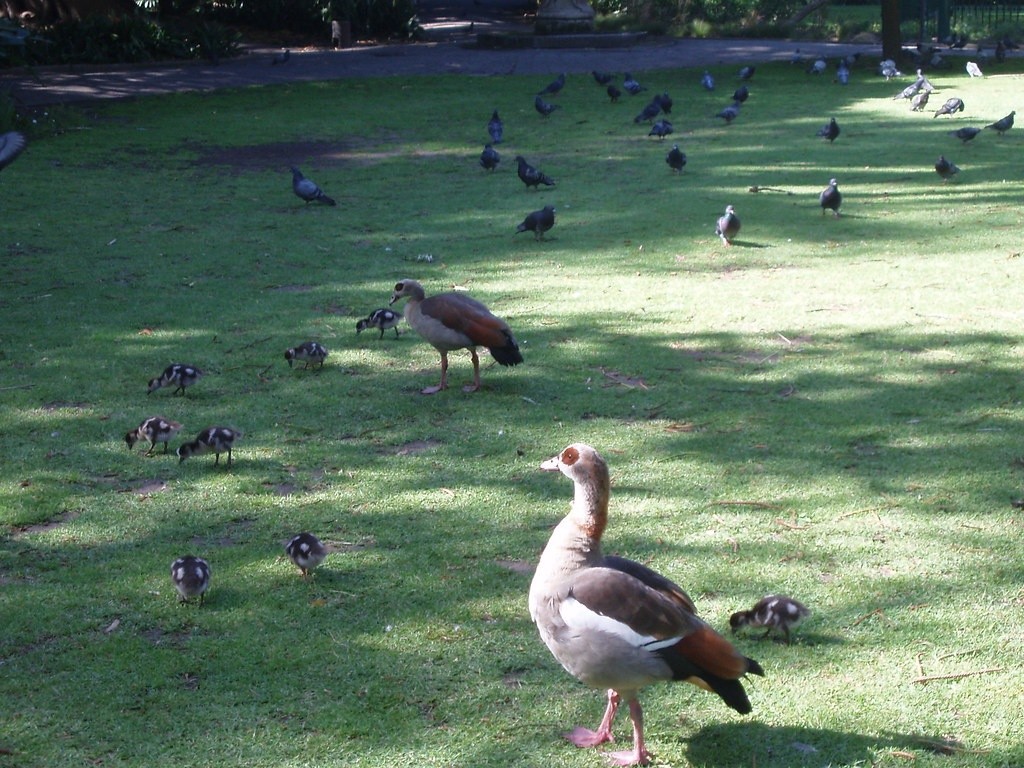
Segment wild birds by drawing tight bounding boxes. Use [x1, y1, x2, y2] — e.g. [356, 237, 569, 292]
[821, 179, 842, 215]
[355, 309, 404, 339]
[171, 554, 211, 608]
[124, 415, 240, 468]
[389, 278, 524, 394]
[489, 110, 503, 141]
[714, 205, 741, 247]
[147, 364, 202, 396]
[535, 27, 1020, 158]
[516, 204, 556, 240]
[465, 22, 474, 32]
[479, 144, 499, 169]
[285, 342, 328, 372]
[272, 50, 289, 65]
[935, 155, 961, 185]
[666, 143, 687, 172]
[528, 443, 809, 766]
[291, 167, 336, 206]
[514, 156, 555, 190]
[284, 532, 328, 579]
